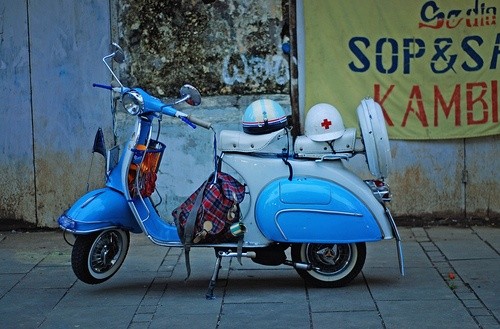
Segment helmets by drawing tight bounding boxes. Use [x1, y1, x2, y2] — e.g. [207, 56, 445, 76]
[242, 98, 288, 135]
[304, 103, 345, 142]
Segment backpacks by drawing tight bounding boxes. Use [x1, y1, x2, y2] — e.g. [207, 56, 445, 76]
[172, 155, 247, 248]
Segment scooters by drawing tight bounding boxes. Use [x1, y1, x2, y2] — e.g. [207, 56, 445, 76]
[57, 42, 405, 287]
